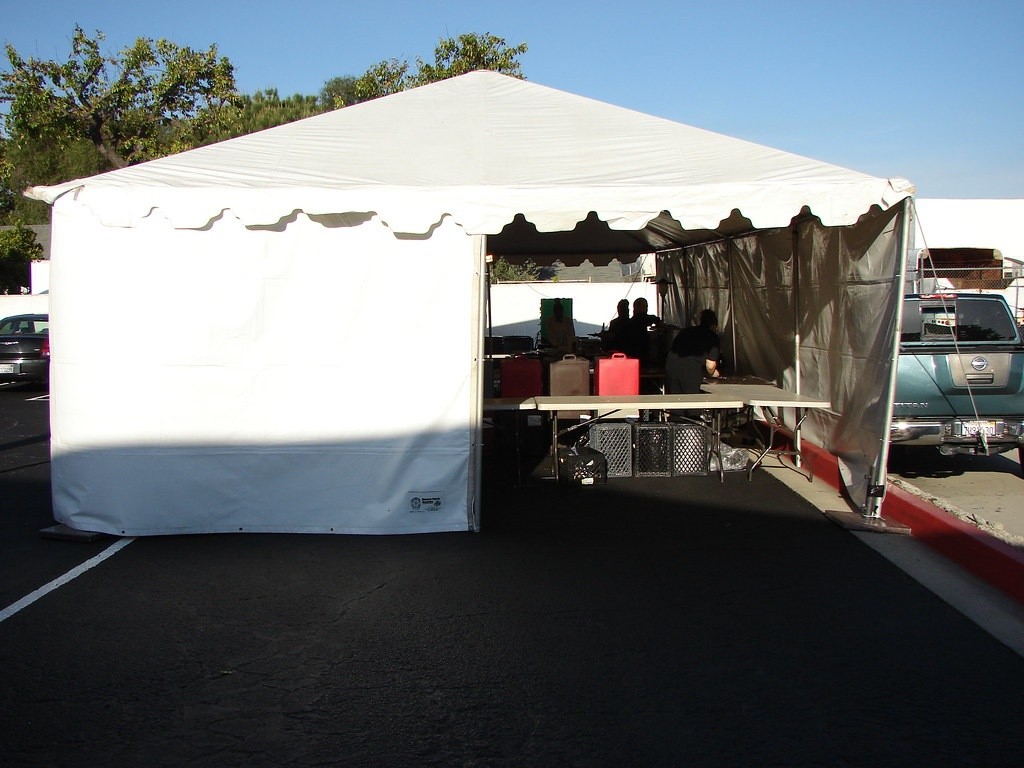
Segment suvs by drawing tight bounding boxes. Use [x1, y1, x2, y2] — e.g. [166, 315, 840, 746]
[890, 293, 1024, 471]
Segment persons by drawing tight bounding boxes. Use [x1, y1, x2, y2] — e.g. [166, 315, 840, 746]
[547, 298, 661, 356]
[665, 308, 722, 394]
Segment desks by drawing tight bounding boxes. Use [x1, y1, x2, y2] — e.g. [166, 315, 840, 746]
[483, 396, 536, 489]
[703, 374, 775, 446]
[590, 368, 668, 394]
[700, 385, 831, 481]
[520, 396, 743, 483]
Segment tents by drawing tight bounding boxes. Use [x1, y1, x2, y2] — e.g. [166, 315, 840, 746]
[20, 69, 916, 532]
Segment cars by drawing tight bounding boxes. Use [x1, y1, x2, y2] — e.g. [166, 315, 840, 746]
[0, 314, 49, 384]
